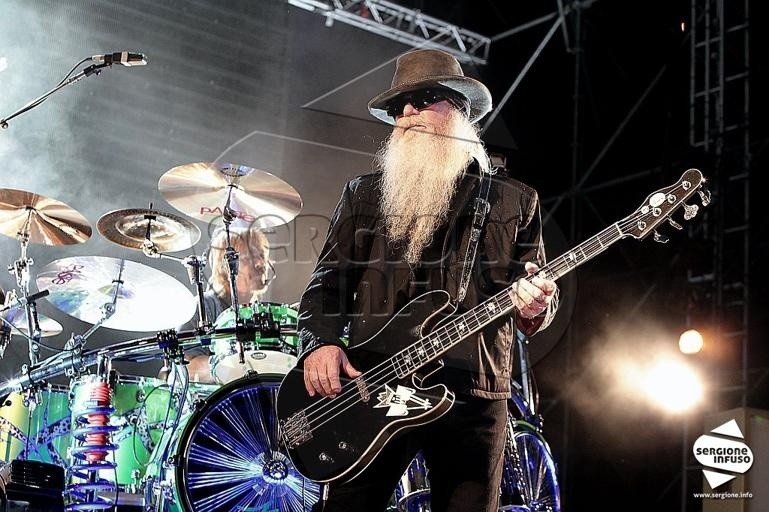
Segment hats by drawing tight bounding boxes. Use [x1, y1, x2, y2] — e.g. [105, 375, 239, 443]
[368, 49, 492, 126]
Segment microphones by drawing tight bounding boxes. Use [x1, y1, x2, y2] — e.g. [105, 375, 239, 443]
[4, 288, 49, 311]
[91, 51, 149, 67]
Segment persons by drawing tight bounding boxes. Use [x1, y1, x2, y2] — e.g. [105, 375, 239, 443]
[175, 228, 270, 385]
[297, 48, 560, 512]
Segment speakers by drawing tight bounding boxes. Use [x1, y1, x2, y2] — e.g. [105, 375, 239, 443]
[701, 406, 769, 512]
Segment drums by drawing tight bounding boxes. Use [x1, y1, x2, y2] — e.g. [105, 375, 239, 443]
[63, 372, 189, 509]
[140, 384, 221, 511]
[208, 300, 300, 384]
[163, 373, 330, 509]
[1, 380, 76, 469]
[394, 458, 430, 506]
[497, 422, 560, 511]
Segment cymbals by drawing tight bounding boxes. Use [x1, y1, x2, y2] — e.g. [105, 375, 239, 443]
[0, 189, 92, 246]
[158, 162, 303, 230]
[96, 208, 200, 253]
[35, 255, 197, 332]
[0, 306, 63, 338]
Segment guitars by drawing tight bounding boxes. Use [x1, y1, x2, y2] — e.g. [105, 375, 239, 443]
[275, 167, 711, 484]
[507, 378, 532, 420]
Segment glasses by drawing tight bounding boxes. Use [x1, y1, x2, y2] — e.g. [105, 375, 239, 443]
[386, 90, 451, 116]
